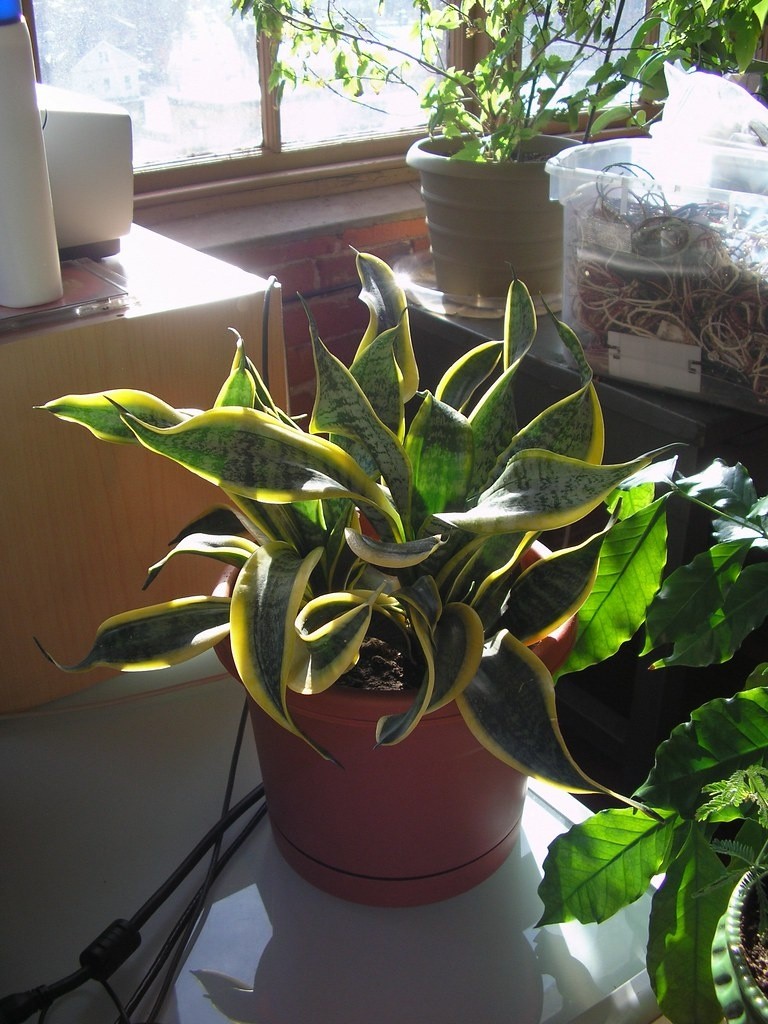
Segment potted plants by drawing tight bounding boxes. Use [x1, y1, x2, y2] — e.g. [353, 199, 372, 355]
[231, 0, 767, 325]
[30, 241, 695, 905]
[534, 451, 768, 1024]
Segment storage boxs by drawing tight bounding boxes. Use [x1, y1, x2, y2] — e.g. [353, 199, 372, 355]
[544, 137, 768, 419]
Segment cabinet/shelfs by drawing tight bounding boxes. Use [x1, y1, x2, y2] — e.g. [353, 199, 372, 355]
[355, 266, 723, 608]
[0, 222, 288, 710]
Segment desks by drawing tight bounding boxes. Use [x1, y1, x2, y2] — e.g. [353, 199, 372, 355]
[0, 679, 669, 1023]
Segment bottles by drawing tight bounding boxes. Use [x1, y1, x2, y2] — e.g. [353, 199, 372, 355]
[1, 0, 63, 310]
